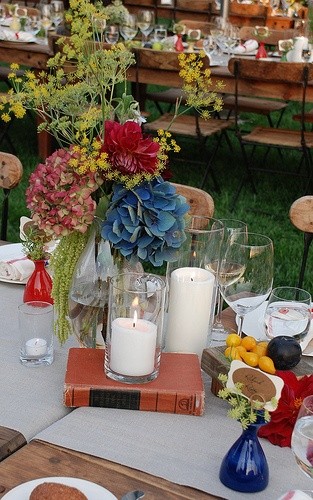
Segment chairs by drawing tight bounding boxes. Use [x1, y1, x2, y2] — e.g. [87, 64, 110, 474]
[0, 152, 23, 241]
[49, 0, 313, 214]
[168, 182, 214, 268]
[289, 195, 313, 301]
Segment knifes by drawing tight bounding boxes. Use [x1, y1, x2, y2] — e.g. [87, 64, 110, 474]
[120, 489, 144, 500]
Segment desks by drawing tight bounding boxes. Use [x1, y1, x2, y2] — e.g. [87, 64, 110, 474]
[0, 40, 55, 162]
[0, 242, 313, 500]
[63, 54, 313, 102]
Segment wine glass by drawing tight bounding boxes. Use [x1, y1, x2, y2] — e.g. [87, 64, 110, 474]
[138, 9, 156, 46]
[40, 8, 52, 44]
[50, 1, 63, 29]
[208, 17, 241, 65]
[204, 219, 249, 342]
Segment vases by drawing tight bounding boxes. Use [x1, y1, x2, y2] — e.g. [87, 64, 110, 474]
[280, 51, 288, 62]
[176, 34, 184, 51]
[256, 42, 266, 60]
[219, 413, 271, 493]
[23, 261, 55, 307]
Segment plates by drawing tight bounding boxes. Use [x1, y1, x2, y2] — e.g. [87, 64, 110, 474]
[0, 241, 58, 284]
[235, 301, 313, 357]
[0, 476, 117, 500]
[0, 31, 32, 41]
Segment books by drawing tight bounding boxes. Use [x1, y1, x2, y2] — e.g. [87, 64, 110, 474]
[63, 347, 205, 418]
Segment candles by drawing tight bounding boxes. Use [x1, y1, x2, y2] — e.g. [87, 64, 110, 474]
[26, 339, 47, 356]
[166, 267, 215, 362]
[293, 36, 308, 62]
[110, 310, 158, 376]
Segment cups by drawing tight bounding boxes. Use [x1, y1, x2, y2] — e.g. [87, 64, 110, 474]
[25, 15, 40, 36]
[119, 13, 138, 41]
[217, 232, 274, 338]
[263, 287, 312, 344]
[105, 25, 119, 45]
[291, 395, 313, 480]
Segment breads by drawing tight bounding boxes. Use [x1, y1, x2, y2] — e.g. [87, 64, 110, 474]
[30, 482, 87, 500]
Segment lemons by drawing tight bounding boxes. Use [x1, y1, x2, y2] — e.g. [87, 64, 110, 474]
[239, 336, 276, 375]
[224, 333, 247, 360]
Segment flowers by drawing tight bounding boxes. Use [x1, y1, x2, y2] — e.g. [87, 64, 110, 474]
[217, 372, 278, 430]
[257, 370, 313, 448]
[0, 0, 227, 347]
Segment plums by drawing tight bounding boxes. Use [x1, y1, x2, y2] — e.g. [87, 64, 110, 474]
[267, 336, 302, 370]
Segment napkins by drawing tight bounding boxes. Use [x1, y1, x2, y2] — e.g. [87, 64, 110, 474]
[235, 39, 259, 53]
